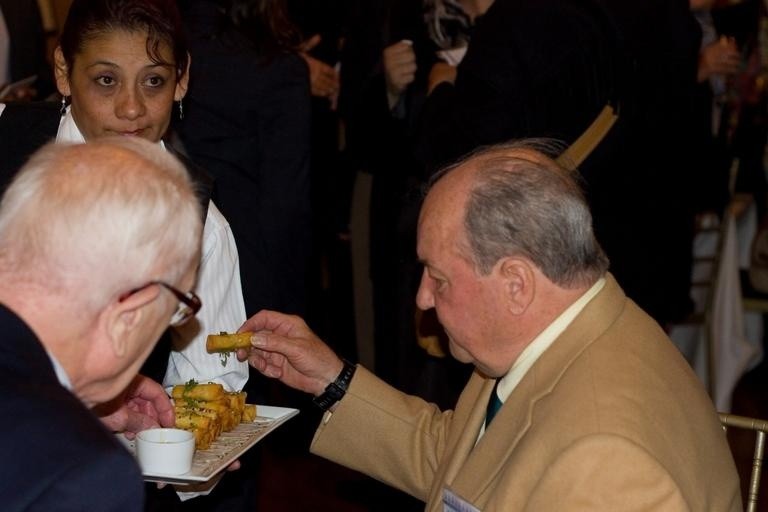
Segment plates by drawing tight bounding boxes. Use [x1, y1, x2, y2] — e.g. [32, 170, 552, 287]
[110, 397, 300, 483]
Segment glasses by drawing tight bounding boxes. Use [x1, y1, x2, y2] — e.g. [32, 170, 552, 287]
[119, 280, 202, 328]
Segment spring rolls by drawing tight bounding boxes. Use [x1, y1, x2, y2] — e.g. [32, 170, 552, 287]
[173, 384, 257, 450]
[205, 331, 256, 353]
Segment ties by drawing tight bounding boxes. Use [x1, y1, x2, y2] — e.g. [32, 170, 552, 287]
[485, 388, 502, 432]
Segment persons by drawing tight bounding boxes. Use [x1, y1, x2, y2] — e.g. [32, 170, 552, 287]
[0, 0, 250, 511]
[179, 2, 766, 511]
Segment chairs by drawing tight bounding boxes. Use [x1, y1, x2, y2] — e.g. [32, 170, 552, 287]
[717, 412, 768, 512]
